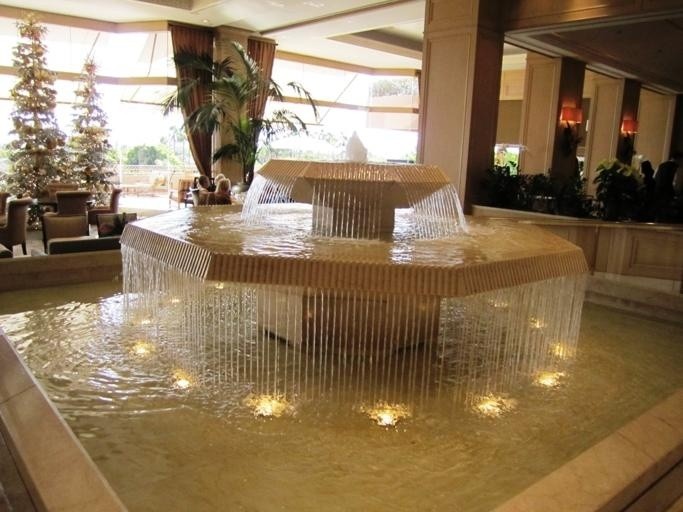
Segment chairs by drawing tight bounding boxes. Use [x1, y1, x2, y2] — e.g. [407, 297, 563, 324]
[0, 182, 137, 258]
[167, 177, 235, 208]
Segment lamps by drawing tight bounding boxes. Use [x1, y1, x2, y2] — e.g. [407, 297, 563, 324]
[561, 107, 583, 135]
[623, 120, 639, 142]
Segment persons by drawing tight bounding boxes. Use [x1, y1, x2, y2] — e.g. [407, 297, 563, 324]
[194, 174, 232, 205]
